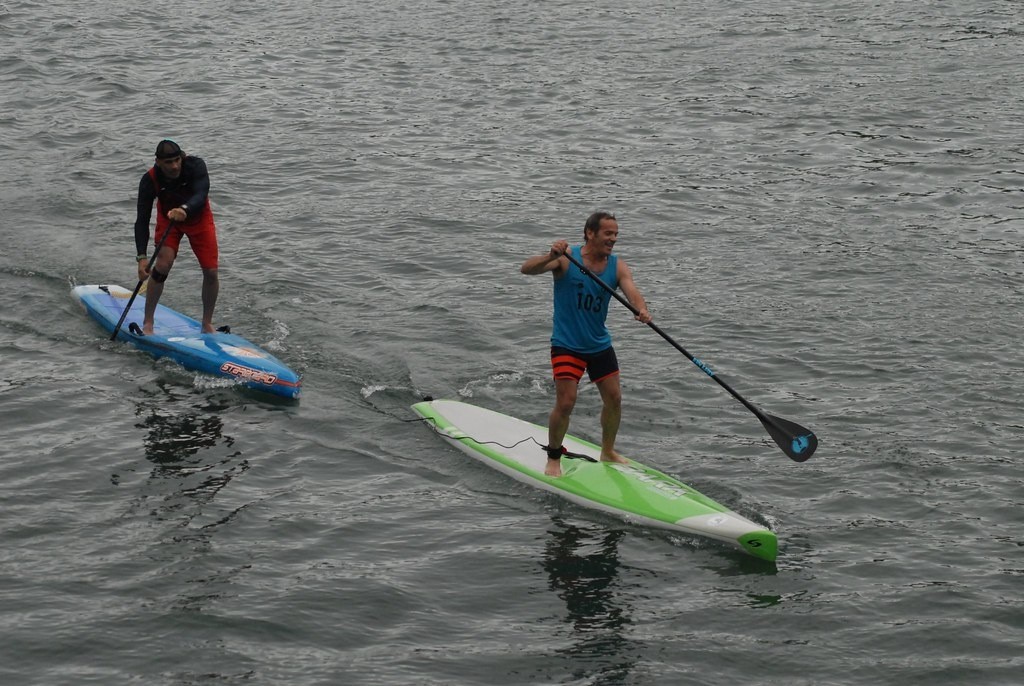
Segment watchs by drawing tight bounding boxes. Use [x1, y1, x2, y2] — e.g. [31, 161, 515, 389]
[136, 256, 147, 262]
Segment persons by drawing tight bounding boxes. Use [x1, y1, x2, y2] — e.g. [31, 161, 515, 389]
[521, 213, 653, 478]
[134, 139, 219, 335]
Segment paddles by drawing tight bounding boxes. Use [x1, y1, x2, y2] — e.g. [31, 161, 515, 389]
[110, 219, 174, 341]
[561, 245, 819, 464]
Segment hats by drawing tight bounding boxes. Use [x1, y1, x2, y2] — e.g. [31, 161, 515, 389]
[155, 140, 181, 160]
